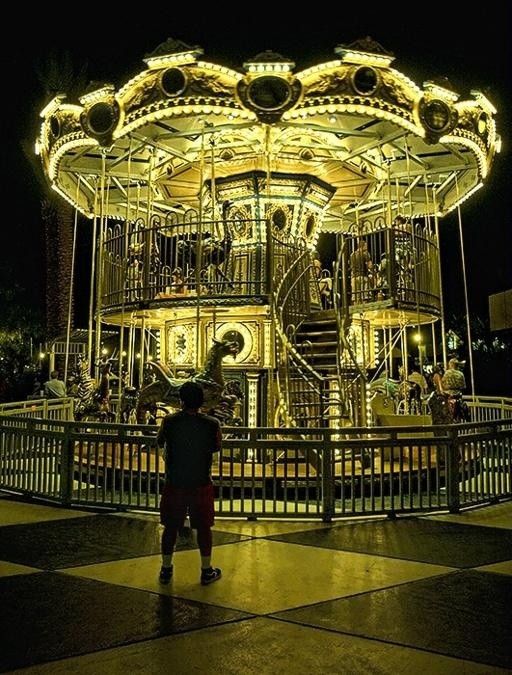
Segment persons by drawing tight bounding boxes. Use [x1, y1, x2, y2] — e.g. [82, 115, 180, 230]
[45, 370, 68, 397]
[441, 355, 467, 423]
[168, 266, 187, 293]
[156, 380, 223, 585]
[65, 376, 79, 394]
[379, 251, 387, 273]
[394, 214, 411, 259]
[350, 240, 371, 302]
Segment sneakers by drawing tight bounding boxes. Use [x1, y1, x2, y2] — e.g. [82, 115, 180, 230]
[202, 565, 222, 584]
[159, 565, 175, 583]
